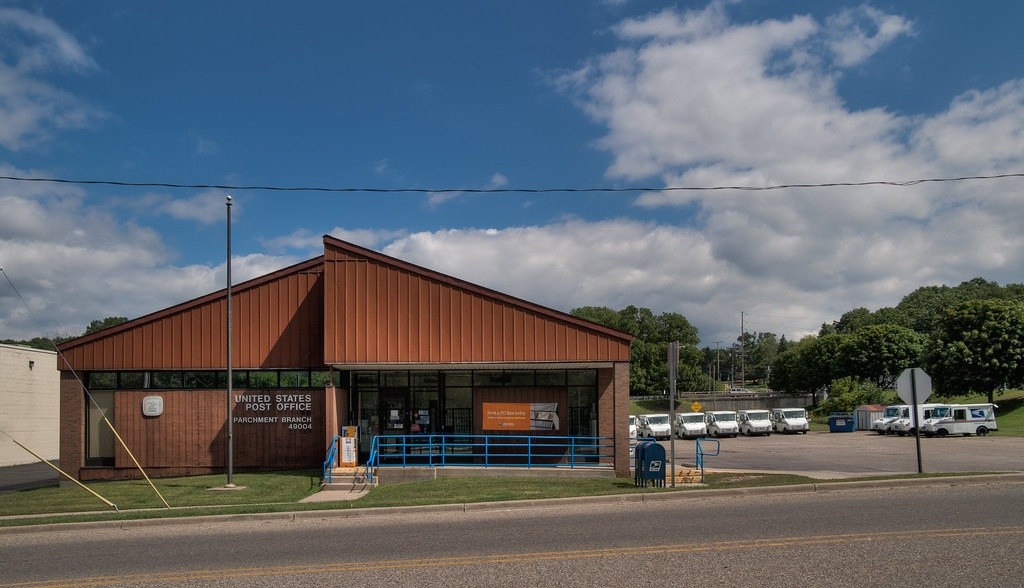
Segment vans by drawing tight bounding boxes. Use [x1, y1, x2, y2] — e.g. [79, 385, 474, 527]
[730, 388, 759, 398]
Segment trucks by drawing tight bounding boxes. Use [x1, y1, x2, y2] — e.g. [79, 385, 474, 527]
[638, 413, 671, 441]
[738, 409, 772, 436]
[873, 404, 999, 437]
[628, 414, 639, 443]
[673, 412, 708, 439]
[706, 411, 739, 438]
[772, 408, 812, 434]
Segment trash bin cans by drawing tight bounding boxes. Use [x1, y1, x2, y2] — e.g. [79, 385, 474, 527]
[641, 442, 667, 489]
[634, 442, 656, 488]
[828, 411, 857, 433]
